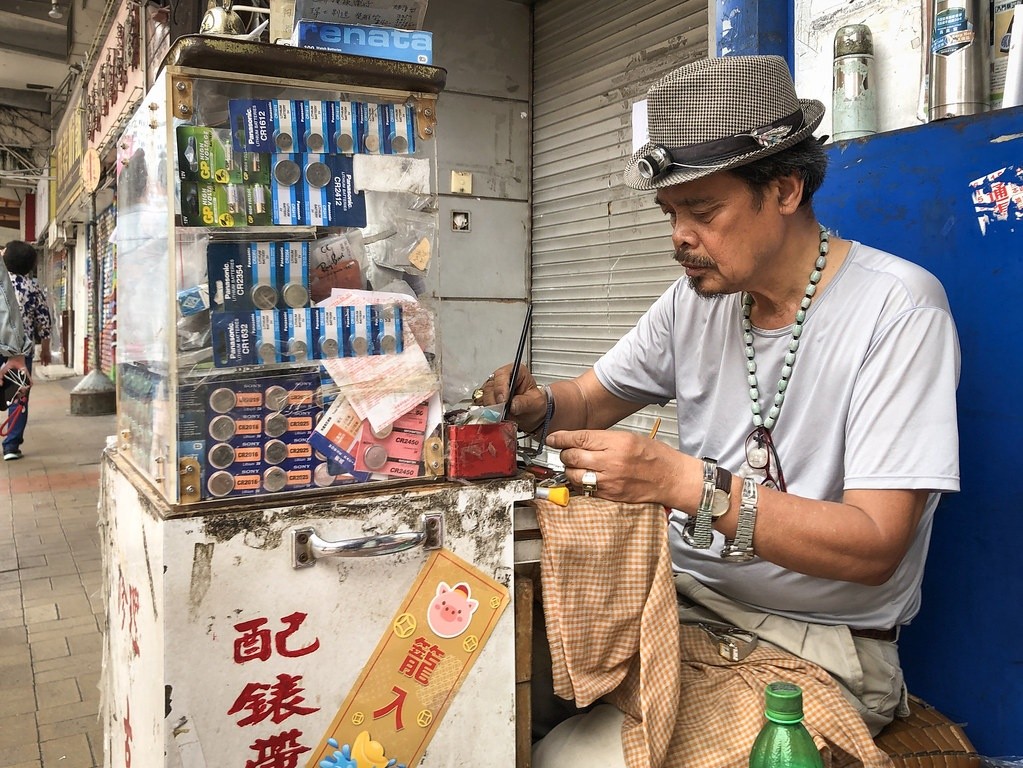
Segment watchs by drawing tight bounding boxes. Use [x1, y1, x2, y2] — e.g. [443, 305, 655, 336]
[699, 622, 759, 661]
[720, 477, 758, 563]
[682, 457, 732, 549]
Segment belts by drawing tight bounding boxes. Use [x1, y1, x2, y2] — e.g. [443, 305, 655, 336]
[850, 625, 899, 645]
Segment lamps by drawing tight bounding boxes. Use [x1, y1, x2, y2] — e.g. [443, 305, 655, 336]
[69, 63, 82, 74]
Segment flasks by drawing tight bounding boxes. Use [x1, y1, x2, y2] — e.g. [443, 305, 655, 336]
[831, 24, 877, 142]
[928, 1, 991, 123]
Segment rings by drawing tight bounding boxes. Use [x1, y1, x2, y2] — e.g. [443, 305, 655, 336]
[582, 469, 597, 497]
[487, 373, 495, 383]
[472, 389, 484, 405]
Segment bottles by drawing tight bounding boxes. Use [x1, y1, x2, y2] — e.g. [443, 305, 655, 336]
[750, 682, 823, 768]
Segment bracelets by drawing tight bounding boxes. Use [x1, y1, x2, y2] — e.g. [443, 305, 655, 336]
[516, 384, 556, 465]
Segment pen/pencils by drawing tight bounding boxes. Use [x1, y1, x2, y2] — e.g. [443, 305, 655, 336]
[498, 304, 534, 423]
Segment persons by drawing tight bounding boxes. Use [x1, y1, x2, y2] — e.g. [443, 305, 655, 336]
[486, 55, 962, 768]
[0, 240, 52, 462]
[116, 146, 172, 403]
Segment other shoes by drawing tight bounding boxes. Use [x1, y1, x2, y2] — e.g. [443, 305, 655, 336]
[3, 450, 23, 460]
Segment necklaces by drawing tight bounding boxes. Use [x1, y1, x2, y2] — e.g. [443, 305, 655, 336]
[736, 222, 828, 493]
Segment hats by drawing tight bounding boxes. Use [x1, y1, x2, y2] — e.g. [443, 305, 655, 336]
[621, 55, 826, 191]
